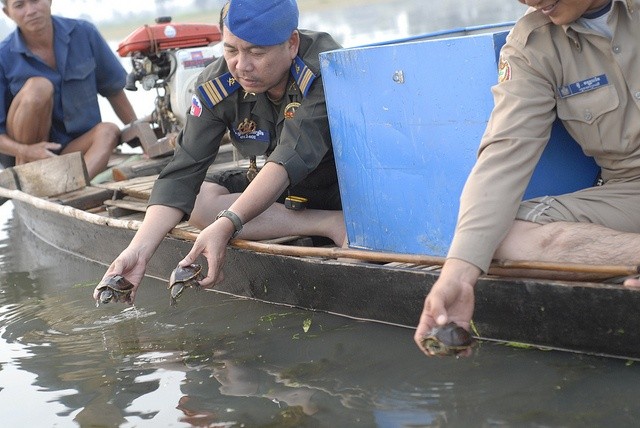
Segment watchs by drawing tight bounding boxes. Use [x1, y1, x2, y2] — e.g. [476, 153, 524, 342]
[215, 210, 244, 238]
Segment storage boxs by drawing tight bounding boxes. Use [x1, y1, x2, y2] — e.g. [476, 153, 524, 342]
[319, 22, 602, 256]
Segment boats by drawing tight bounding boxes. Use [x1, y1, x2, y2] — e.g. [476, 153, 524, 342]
[0, 8, 640, 361]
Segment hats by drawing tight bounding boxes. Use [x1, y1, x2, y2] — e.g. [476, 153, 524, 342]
[221, 0, 299, 46]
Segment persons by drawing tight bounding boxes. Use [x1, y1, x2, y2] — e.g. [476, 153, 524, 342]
[0, 0, 137, 182]
[93, 0, 347, 304]
[414, 1, 639, 358]
